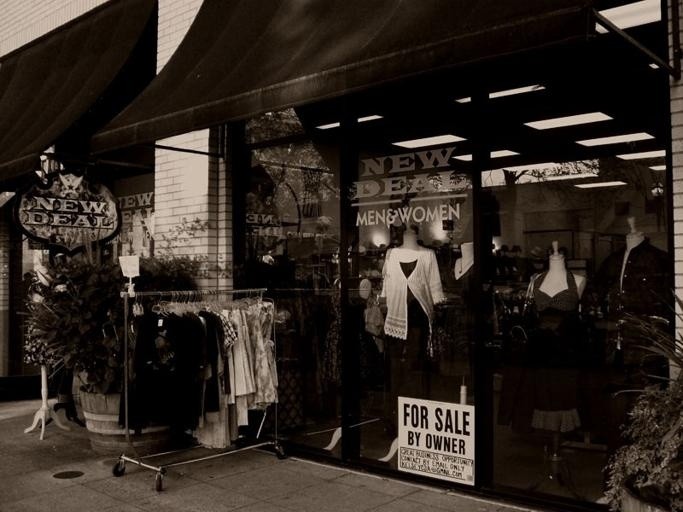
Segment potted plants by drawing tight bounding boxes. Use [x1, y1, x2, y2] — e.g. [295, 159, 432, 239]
[25, 214, 236, 459]
[601, 287, 682, 512]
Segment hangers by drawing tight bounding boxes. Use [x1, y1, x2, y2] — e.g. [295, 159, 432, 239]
[131, 288, 260, 316]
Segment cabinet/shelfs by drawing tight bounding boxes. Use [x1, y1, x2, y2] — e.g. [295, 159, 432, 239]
[111, 289, 287, 492]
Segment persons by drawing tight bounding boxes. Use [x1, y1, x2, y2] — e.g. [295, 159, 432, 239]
[21, 264, 63, 373]
[316, 210, 669, 459]
